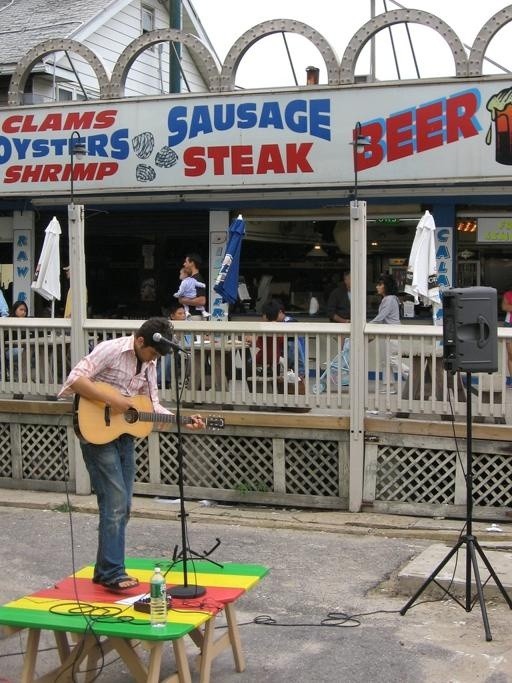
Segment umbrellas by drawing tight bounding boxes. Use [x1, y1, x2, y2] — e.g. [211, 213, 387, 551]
[212, 214, 247, 305]
[401, 208, 443, 326]
[30, 216, 63, 318]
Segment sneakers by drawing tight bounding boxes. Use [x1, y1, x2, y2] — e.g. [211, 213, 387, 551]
[378, 385, 397, 394]
[202, 311, 213, 318]
[186, 312, 192, 322]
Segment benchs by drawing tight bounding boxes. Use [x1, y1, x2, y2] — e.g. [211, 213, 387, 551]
[247, 369, 293, 411]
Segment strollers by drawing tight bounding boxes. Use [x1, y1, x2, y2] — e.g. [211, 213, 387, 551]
[310, 335, 348, 394]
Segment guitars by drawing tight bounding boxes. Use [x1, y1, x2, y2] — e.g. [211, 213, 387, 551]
[72, 381, 225, 446]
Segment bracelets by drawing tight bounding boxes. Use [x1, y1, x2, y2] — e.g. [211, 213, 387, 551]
[173, 337, 182, 344]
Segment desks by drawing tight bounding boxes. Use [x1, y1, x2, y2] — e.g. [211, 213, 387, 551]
[55, 556, 273, 682]
[5, 335, 98, 401]
[0, 587, 225, 682]
[397, 346, 467, 421]
[182, 342, 252, 410]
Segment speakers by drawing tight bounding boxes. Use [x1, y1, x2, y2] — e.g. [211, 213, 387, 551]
[443, 286, 498, 372]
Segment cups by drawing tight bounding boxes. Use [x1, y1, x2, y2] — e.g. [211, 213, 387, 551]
[196, 335, 201, 342]
[494, 99, 511, 167]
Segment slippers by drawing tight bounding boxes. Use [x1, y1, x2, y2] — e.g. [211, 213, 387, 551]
[92, 574, 142, 589]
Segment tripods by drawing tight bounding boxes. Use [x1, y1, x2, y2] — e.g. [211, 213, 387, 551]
[400, 371, 512, 641]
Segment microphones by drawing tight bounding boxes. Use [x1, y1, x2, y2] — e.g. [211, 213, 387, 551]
[152, 333, 188, 354]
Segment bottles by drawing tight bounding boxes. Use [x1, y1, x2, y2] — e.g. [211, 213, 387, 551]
[149, 567, 167, 628]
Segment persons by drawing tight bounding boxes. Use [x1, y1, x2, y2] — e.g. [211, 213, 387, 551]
[366, 272, 411, 394]
[180, 251, 208, 321]
[172, 268, 211, 318]
[5, 299, 33, 381]
[275, 299, 307, 385]
[0, 290, 10, 317]
[501, 289, 512, 387]
[158, 304, 191, 388]
[246, 298, 283, 377]
[322, 270, 350, 322]
[53, 317, 183, 591]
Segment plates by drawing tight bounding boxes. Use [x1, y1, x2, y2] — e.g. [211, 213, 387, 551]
[194, 340, 210, 344]
[228, 341, 243, 345]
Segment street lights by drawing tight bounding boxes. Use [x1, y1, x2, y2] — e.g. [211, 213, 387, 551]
[348, 119, 372, 202]
[69, 130, 86, 206]
